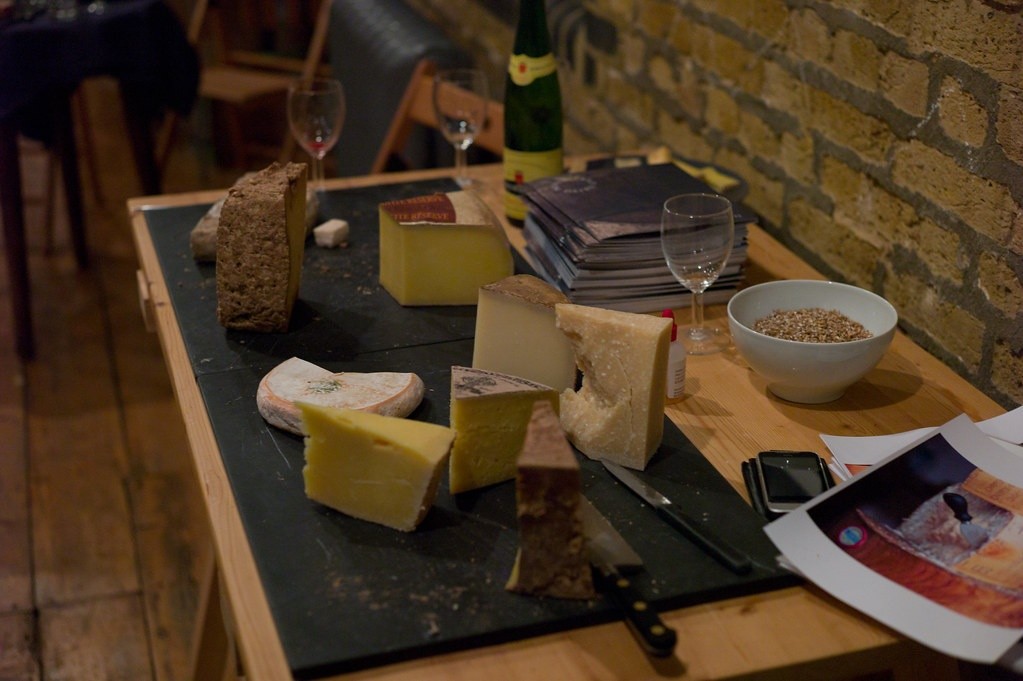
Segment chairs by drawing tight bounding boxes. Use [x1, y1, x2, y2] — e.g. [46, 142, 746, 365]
[152, 1, 339, 193]
[370, 55, 508, 178]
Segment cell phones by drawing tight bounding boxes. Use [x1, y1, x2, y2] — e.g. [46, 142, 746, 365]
[757, 451, 829, 516]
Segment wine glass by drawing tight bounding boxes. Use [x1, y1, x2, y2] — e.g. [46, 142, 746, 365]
[285, 76, 343, 212]
[658, 193, 734, 355]
[433, 71, 492, 201]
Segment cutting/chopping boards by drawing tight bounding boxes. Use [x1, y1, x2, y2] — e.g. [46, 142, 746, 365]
[142, 177, 541, 380]
[195, 337, 806, 681]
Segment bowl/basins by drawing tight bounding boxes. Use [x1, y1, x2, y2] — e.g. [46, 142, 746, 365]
[726, 279, 897, 403]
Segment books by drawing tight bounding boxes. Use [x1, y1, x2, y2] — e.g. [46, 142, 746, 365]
[521, 159, 760, 315]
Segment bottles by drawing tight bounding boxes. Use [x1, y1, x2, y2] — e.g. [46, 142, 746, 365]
[503, 1, 564, 226]
[663, 309, 688, 403]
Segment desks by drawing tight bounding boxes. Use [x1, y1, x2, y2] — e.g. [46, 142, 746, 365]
[1, 1, 203, 369]
[126, 147, 1013, 681]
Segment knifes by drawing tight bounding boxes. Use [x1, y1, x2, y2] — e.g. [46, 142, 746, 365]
[581, 494, 679, 660]
[603, 460, 753, 574]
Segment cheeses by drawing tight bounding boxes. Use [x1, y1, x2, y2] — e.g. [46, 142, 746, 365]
[188, 160, 674, 601]
